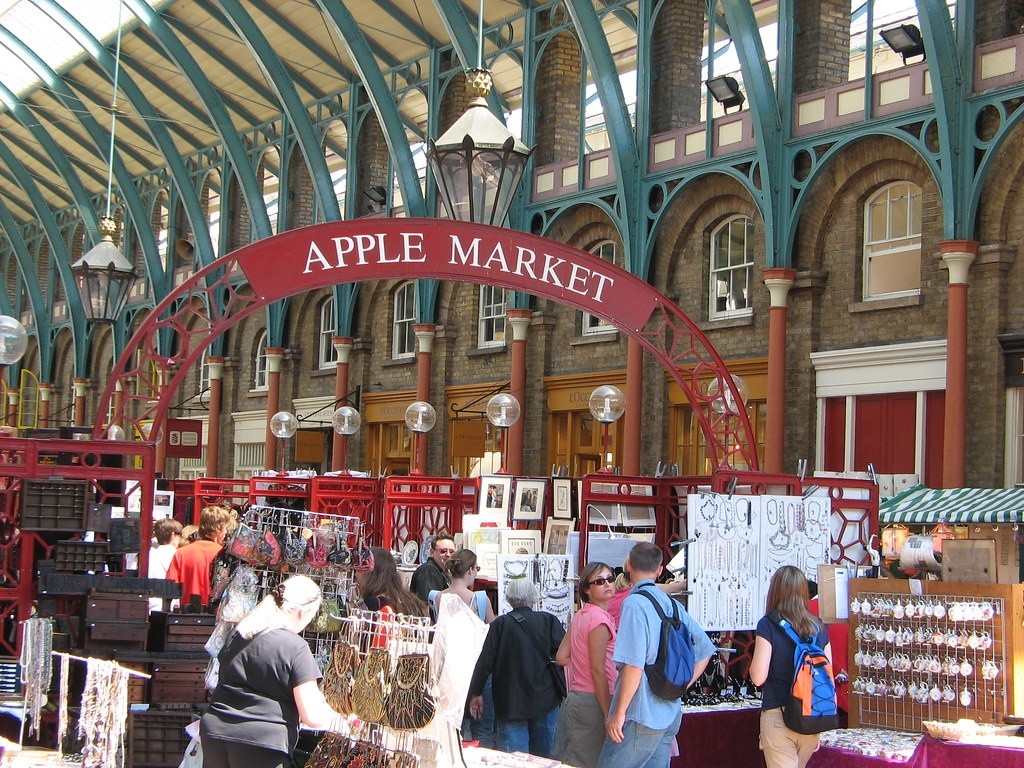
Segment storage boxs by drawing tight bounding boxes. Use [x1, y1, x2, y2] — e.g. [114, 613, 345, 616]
[476, 474, 513, 527]
[0, 475, 218, 768]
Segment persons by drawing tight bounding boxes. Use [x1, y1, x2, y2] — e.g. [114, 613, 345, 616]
[606, 555, 686, 636]
[522, 489, 537, 512]
[551, 562, 620, 768]
[749, 566, 837, 768]
[303, 533, 495, 768]
[126, 504, 240, 615]
[470, 578, 568, 760]
[200, 575, 349, 768]
[596, 542, 714, 768]
[487, 485, 502, 509]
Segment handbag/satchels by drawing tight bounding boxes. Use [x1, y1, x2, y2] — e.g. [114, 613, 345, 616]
[325, 615, 355, 716]
[384, 624, 437, 734]
[329, 532, 352, 573]
[305, 531, 327, 569]
[227, 509, 266, 566]
[352, 536, 375, 573]
[548, 658, 569, 699]
[351, 621, 385, 724]
[280, 522, 307, 566]
[253, 516, 285, 570]
[306, 582, 342, 633]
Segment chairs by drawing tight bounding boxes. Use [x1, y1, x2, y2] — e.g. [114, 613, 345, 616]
[0, 617, 56, 753]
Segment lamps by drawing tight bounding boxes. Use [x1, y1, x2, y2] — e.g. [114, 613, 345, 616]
[362, 186, 386, 207]
[0, 315, 28, 364]
[704, 75, 746, 115]
[879, 23, 927, 65]
[424, 0, 538, 229]
[104, 369, 749, 441]
[72, 0, 137, 323]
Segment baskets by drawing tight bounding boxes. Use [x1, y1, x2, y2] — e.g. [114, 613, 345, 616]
[920, 718, 1021, 742]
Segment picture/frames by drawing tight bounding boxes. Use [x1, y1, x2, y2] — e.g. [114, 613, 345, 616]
[578, 480, 618, 526]
[500, 529, 541, 554]
[469, 526, 511, 583]
[542, 515, 576, 556]
[550, 476, 572, 519]
[620, 483, 656, 526]
[512, 478, 547, 521]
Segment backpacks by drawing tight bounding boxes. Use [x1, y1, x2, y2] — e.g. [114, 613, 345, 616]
[636, 588, 696, 701]
[763, 610, 842, 734]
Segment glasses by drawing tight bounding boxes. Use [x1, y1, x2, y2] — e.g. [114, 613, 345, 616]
[472, 566, 481, 571]
[588, 573, 617, 586]
[433, 548, 456, 554]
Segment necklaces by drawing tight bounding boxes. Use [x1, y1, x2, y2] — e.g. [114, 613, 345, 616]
[693, 498, 829, 628]
[502, 554, 571, 633]
[57, 655, 137, 768]
[20, 616, 53, 739]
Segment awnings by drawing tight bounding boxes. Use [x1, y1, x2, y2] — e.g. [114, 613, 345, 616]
[867, 484, 1024, 526]
